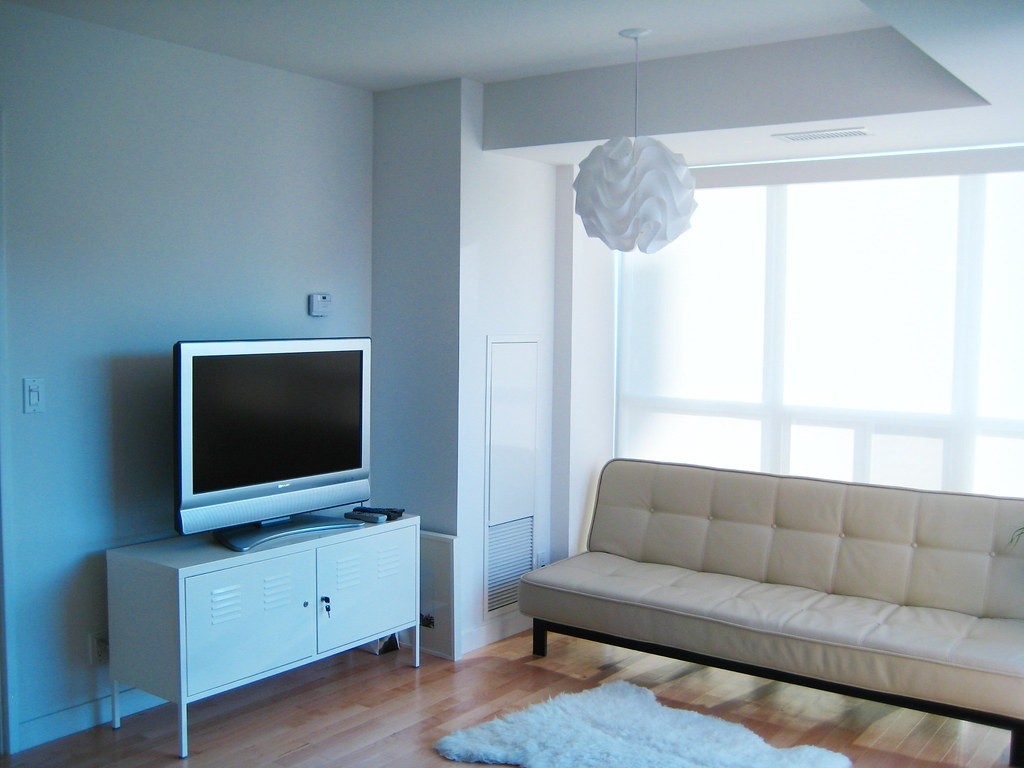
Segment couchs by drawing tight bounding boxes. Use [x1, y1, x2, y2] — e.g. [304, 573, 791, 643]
[517, 457, 1024, 768]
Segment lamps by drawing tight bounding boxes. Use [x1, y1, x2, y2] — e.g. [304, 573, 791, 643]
[571, 27, 699, 254]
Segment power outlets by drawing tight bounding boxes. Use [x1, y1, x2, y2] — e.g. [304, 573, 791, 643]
[88, 628, 109, 667]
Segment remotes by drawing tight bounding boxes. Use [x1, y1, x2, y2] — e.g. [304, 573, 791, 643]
[344, 506, 405, 523]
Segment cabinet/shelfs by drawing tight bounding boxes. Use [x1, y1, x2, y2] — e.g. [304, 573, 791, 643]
[106, 509, 421, 758]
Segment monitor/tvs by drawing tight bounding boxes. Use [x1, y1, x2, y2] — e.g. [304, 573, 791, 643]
[173, 336, 370, 552]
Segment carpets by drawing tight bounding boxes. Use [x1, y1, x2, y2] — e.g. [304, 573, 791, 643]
[437, 680, 853, 768]
